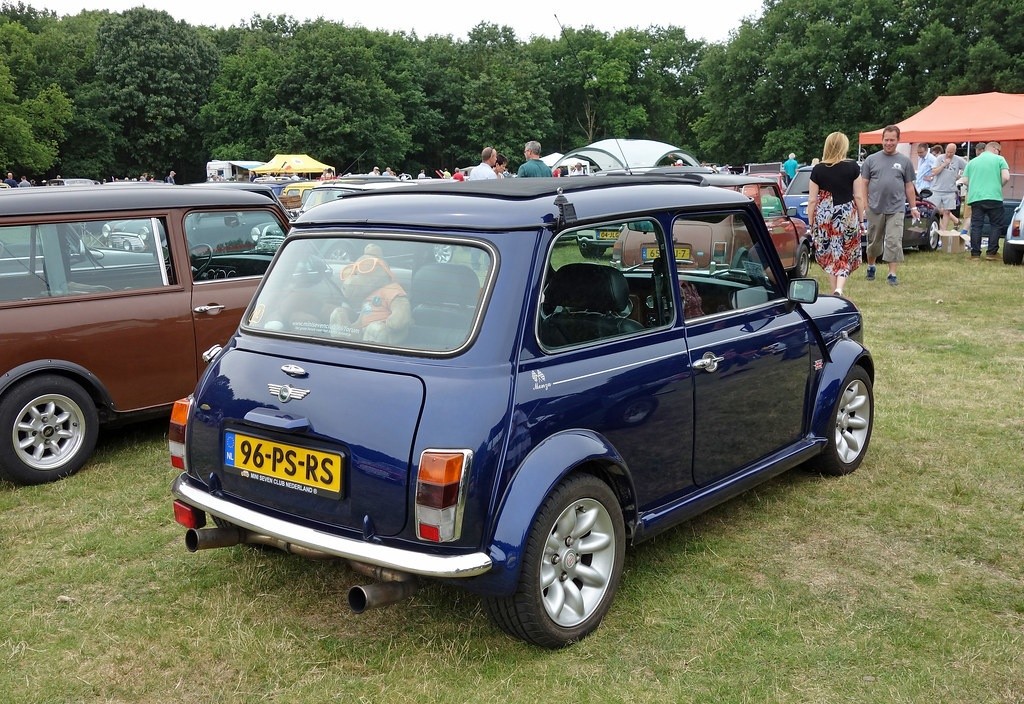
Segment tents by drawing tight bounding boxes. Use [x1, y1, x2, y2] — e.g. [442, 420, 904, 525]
[858, 93, 1024, 202]
[250, 153, 335, 181]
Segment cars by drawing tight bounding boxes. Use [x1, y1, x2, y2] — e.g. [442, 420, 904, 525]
[554, 160, 943, 279]
[1003, 194, 1024, 264]
[0, 175, 456, 486]
[167, 169, 877, 652]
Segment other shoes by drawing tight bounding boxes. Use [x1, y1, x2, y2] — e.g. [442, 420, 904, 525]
[834, 289, 841, 296]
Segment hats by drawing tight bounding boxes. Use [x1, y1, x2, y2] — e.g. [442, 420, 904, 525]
[374, 167, 379, 170]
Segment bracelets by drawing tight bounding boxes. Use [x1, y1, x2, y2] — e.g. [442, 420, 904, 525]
[860, 221, 864, 223]
[910, 207, 917, 210]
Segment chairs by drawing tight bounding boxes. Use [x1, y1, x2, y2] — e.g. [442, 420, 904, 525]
[541, 264, 644, 346]
[67, 281, 113, 294]
[411, 263, 479, 330]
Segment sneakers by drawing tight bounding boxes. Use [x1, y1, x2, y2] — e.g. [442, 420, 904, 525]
[953, 219, 962, 231]
[887, 274, 898, 285]
[968, 256, 980, 261]
[986, 253, 1003, 260]
[866, 267, 875, 280]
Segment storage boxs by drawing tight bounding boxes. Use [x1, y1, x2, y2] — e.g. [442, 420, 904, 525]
[935, 228, 970, 253]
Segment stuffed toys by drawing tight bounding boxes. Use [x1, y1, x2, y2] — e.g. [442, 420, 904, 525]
[325, 246, 413, 344]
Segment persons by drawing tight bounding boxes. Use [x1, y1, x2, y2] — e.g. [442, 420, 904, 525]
[702, 161, 730, 173]
[4, 172, 47, 188]
[811, 158, 819, 166]
[470, 146, 511, 181]
[807, 132, 867, 298]
[553, 163, 588, 177]
[962, 142, 1010, 261]
[370, 166, 394, 177]
[250, 171, 300, 182]
[418, 170, 425, 179]
[400, 173, 406, 180]
[115, 169, 176, 185]
[784, 152, 798, 186]
[861, 124, 921, 285]
[674, 159, 683, 166]
[517, 141, 552, 177]
[916, 142, 986, 232]
[208, 173, 226, 181]
[320, 168, 333, 181]
[442, 166, 464, 182]
